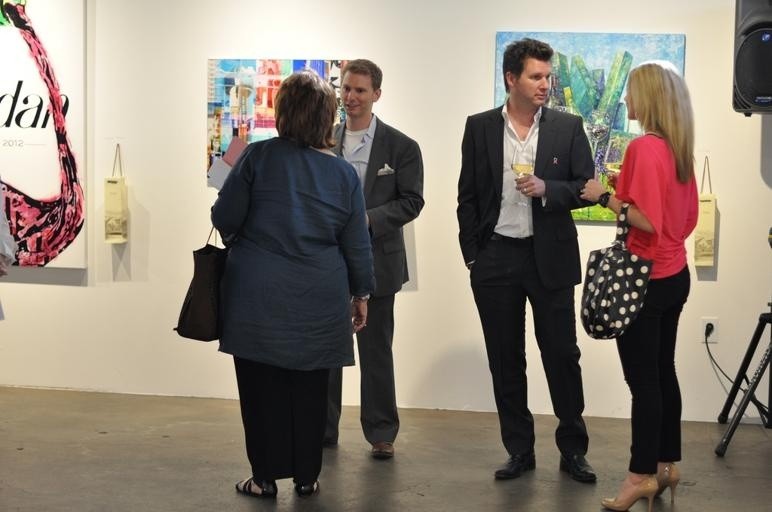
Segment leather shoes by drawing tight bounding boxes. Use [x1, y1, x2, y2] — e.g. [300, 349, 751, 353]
[367, 437, 397, 461]
[493, 450, 538, 481]
[557, 453, 598, 483]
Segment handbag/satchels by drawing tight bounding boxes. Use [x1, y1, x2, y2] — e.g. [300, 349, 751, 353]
[172, 223, 227, 344]
[580, 202, 655, 341]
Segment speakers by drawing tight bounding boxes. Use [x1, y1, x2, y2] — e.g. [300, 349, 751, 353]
[733, 0, 771, 116]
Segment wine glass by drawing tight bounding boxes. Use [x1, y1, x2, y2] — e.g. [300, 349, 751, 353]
[511, 143, 535, 208]
[604, 160, 619, 197]
[579, 110, 610, 163]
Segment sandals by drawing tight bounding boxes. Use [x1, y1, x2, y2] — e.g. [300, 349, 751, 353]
[293, 477, 323, 499]
[232, 473, 280, 500]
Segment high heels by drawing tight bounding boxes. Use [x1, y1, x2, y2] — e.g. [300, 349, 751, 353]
[652, 458, 684, 507]
[599, 468, 658, 511]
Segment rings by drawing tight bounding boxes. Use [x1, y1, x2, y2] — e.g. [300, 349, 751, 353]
[524, 188, 526, 193]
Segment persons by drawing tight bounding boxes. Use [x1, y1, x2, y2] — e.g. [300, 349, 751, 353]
[211, 69, 375, 499]
[323, 59, 424, 460]
[580, 62, 698, 512]
[456, 38, 597, 483]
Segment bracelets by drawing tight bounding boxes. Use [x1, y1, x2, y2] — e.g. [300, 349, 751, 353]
[599, 192, 610, 207]
[353, 294, 371, 302]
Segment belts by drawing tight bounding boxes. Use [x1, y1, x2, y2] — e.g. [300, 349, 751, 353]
[490, 229, 536, 248]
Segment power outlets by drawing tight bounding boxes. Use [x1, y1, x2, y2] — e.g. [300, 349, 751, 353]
[700, 315, 720, 345]
